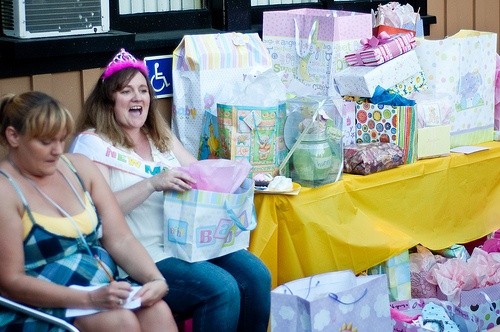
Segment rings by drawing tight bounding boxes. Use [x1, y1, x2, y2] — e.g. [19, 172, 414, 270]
[119, 299, 123, 304]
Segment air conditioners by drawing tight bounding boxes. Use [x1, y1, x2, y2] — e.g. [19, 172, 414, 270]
[0, 0, 111, 39]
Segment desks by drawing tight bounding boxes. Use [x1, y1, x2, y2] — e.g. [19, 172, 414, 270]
[249, 140, 500, 285]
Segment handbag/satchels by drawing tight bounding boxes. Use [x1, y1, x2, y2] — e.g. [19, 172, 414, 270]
[214, 100, 289, 184]
[162, 158, 258, 263]
[268, 269, 393, 331]
[171, 33, 273, 157]
[436, 281, 500, 332]
[413, 28, 497, 149]
[261, 7, 373, 98]
[354, 85, 418, 163]
[381, 17, 425, 38]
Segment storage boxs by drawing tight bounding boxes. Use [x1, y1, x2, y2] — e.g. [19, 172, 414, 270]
[334, 49, 421, 102]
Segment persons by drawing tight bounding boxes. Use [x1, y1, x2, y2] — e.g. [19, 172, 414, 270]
[0, 91, 177, 332]
[69, 48, 272, 332]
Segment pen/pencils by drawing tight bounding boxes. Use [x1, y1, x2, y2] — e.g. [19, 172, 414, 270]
[93, 253, 115, 286]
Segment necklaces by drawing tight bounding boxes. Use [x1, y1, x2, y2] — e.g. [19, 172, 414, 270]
[8, 157, 100, 260]
